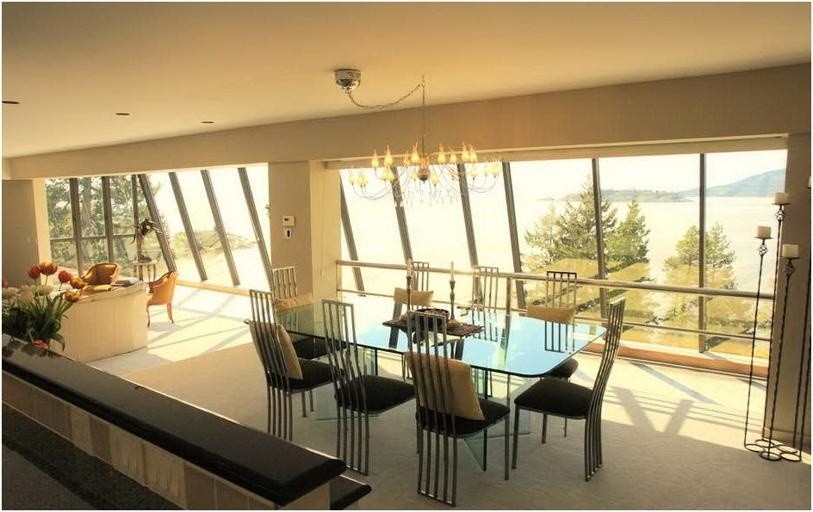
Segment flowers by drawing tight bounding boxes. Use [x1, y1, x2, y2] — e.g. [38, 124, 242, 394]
[3, 261, 87, 353]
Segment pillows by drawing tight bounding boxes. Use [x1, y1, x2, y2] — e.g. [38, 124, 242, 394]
[248, 319, 303, 382]
[394, 288, 433, 306]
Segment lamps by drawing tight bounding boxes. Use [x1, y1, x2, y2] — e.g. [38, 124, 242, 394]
[335, 68, 501, 209]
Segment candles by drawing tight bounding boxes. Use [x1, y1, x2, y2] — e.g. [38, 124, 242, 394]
[756, 177, 811, 258]
[405, 258, 412, 276]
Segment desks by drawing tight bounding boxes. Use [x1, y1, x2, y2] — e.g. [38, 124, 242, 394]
[273, 296, 608, 481]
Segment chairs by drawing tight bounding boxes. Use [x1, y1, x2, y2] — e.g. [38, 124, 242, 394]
[409, 262, 578, 444]
[146, 270, 176, 325]
[321, 298, 420, 475]
[405, 310, 510, 508]
[270, 266, 347, 411]
[512, 296, 626, 481]
[77, 262, 120, 297]
[249, 289, 349, 442]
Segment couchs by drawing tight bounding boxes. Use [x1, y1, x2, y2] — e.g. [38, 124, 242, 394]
[47, 278, 148, 361]
